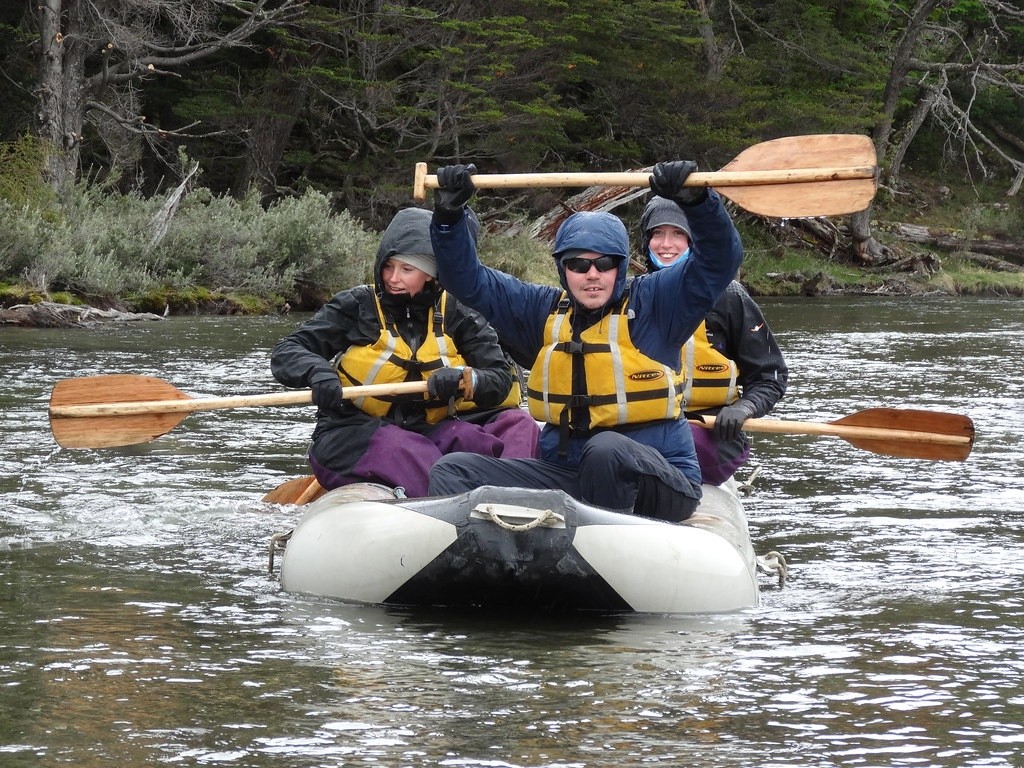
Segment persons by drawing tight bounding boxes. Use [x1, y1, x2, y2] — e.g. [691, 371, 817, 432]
[637, 192, 789, 487]
[427, 159, 745, 524]
[269, 200, 541, 497]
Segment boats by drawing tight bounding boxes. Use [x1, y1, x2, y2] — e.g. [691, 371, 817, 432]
[48, 133, 976, 613]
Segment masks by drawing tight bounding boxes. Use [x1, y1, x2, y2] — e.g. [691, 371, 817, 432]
[649, 245, 691, 270]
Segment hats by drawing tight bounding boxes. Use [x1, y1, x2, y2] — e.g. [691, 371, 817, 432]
[559, 250, 584, 271]
[644, 195, 693, 243]
[389, 253, 438, 279]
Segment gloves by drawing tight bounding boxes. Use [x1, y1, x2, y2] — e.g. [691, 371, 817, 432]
[311, 379, 342, 411]
[647, 159, 698, 200]
[428, 367, 463, 403]
[713, 397, 758, 445]
[434, 164, 479, 225]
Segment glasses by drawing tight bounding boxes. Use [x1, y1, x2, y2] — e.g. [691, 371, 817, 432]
[563, 254, 619, 273]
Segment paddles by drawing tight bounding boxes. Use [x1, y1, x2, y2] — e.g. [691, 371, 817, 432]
[413, 135, 883, 221]
[261, 475, 328, 506]
[46, 366, 478, 450]
[679, 407, 979, 462]
